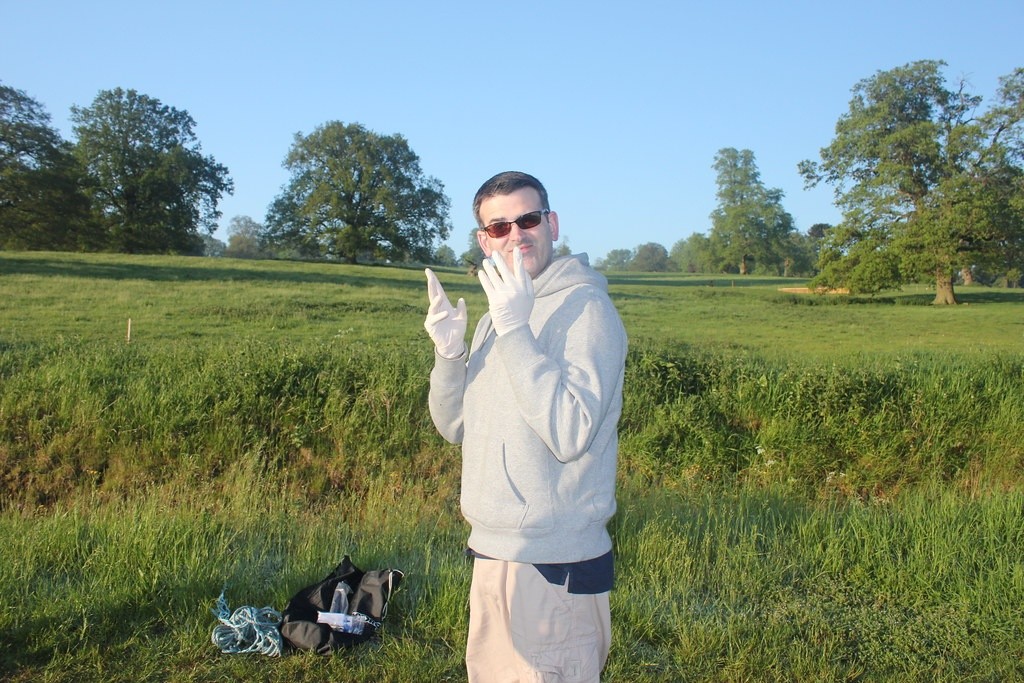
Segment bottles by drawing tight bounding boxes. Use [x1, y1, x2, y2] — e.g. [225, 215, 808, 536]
[325, 614, 365, 636]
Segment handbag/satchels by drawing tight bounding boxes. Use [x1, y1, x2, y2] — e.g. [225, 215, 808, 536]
[279, 554, 404, 656]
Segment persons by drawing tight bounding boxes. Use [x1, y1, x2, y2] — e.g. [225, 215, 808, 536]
[422, 171, 630, 683]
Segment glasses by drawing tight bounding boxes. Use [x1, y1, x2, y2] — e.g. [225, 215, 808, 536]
[481, 209, 550, 238]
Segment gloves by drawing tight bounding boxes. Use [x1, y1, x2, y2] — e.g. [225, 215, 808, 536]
[478, 247, 535, 338]
[424, 268, 467, 359]
